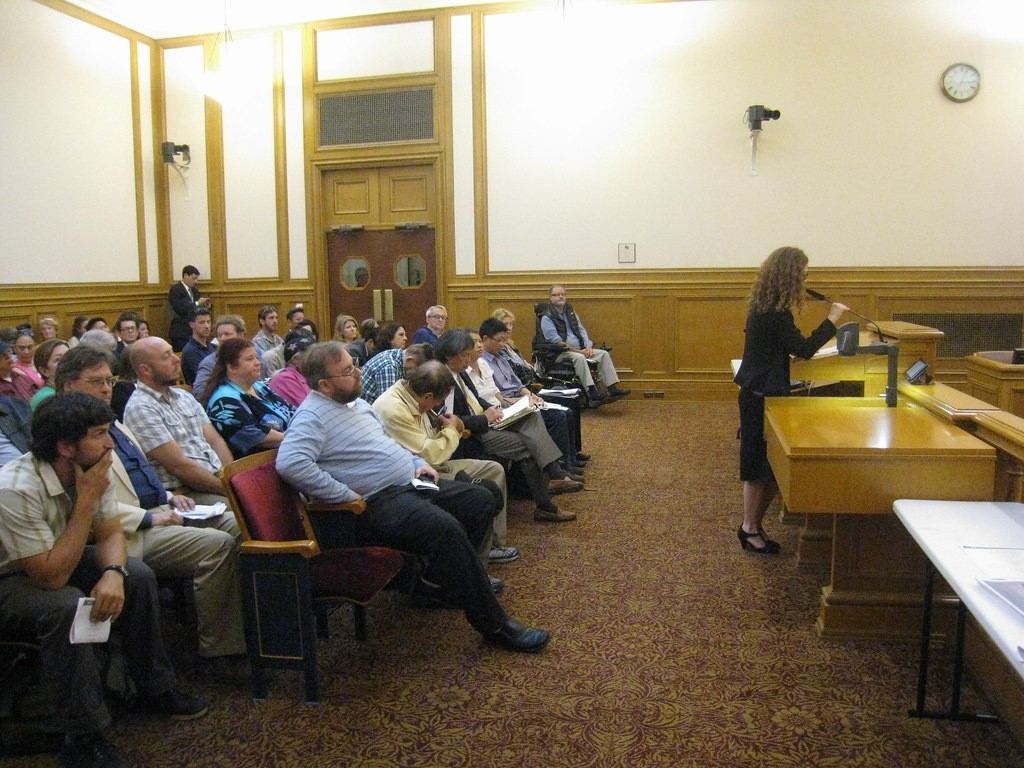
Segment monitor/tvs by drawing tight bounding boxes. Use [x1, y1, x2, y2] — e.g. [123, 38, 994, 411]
[1012, 349, 1024, 364]
[905, 358, 930, 383]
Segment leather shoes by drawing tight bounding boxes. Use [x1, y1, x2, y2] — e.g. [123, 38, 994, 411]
[412, 577, 461, 607]
[482, 619, 551, 650]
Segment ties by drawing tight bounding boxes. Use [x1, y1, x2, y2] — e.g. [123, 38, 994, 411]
[457, 375, 483, 416]
[188, 288, 194, 298]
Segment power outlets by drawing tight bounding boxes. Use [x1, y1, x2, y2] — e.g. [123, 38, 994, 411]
[643, 392, 665, 398]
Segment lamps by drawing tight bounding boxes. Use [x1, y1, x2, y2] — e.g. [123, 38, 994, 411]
[161, 143, 192, 166]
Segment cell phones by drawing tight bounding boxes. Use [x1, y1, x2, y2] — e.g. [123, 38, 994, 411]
[419, 476, 435, 484]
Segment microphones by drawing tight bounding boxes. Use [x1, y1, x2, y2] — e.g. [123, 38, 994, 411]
[806, 289, 887, 344]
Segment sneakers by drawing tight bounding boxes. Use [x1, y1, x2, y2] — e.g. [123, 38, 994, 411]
[117, 688, 209, 719]
[62, 733, 128, 768]
[488, 575, 502, 592]
[488, 545, 520, 564]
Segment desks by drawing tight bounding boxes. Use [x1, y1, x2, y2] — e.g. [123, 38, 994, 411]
[763, 395, 995, 641]
[893, 498, 1024, 722]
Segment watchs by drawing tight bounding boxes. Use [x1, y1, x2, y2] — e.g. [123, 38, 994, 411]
[103, 564, 129, 578]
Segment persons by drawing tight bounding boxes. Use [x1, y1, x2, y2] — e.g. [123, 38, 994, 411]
[492, 308, 591, 461]
[296, 320, 320, 342]
[732, 246, 850, 556]
[0, 392, 210, 767]
[347, 325, 379, 366]
[467, 329, 585, 482]
[478, 317, 587, 474]
[373, 361, 519, 592]
[277, 340, 552, 653]
[287, 308, 306, 332]
[168, 264, 213, 353]
[333, 314, 360, 343]
[360, 319, 379, 337]
[198, 337, 297, 457]
[0, 310, 150, 465]
[251, 307, 284, 360]
[55, 336, 276, 685]
[259, 327, 316, 378]
[361, 342, 435, 405]
[434, 328, 586, 521]
[180, 307, 248, 401]
[541, 285, 631, 406]
[360, 320, 408, 366]
[410, 305, 447, 342]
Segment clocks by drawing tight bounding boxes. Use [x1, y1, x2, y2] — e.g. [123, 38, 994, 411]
[941, 62, 981, 102]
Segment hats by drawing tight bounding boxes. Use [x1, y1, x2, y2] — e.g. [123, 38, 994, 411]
[284, 337, 313, 361]
[0, 338, 11, 353]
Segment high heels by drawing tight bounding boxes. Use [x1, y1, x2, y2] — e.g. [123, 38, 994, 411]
[737, 523, 782, 555]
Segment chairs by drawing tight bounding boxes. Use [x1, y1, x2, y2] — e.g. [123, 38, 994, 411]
[0, 350, 412, 750]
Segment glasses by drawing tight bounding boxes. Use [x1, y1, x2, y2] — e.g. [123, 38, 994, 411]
[192, 307, 206, 318]
[262, 307, 276, 316]
[121, 327, 138, 332]
[486, 334, 506, 343]
[319, 357, 359, 379]
[429, 314, 446, 320]
[79, 376, 119, 387]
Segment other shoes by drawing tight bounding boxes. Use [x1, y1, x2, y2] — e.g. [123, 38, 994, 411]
[547, 479, 583, 494]
[571, 467, 584, 474]
[577, 461, 586, 466]
[576, 453, 591, 460]
[533, 507, 577, 522]
[572, 476, 584, 481]
[591, 392, 608, 400]
[608, 388, 631, 396]
